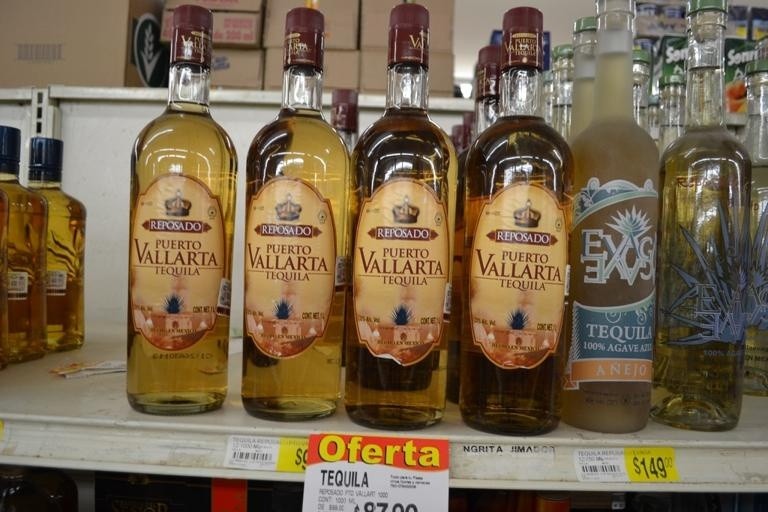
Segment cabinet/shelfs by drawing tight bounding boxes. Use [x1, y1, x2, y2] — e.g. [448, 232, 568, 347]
[0, 80, 767, 493]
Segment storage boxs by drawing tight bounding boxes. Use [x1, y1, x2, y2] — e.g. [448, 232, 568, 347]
[362, 47, 455, 98]
[161, 0, 262, 45]
[262, 1, 359, 48]
[360, 0, 455, 50]
[0, 1, 161, 88]
[210, 49, 263, 89]
[264, 48, 361, 92]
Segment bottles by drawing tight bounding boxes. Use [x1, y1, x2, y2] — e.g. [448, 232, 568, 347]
[26, 137, 86, 352]
[560, 1, 660, 435]
[345, 4, 458, 432]
[330, 90, 360, 365]
[0, 465, 77, 512]
[741, 61, 768, 396]
[0, 189, 9, 371]
[460, 7, 570, 437]
[1, 125, 46, 365]
[650, 1, 751, 432]
[127, 5, 238, 416]
[446, 47, 507, 406]
[451, 4, 767, 155]
[242, 6, 352, 421]
